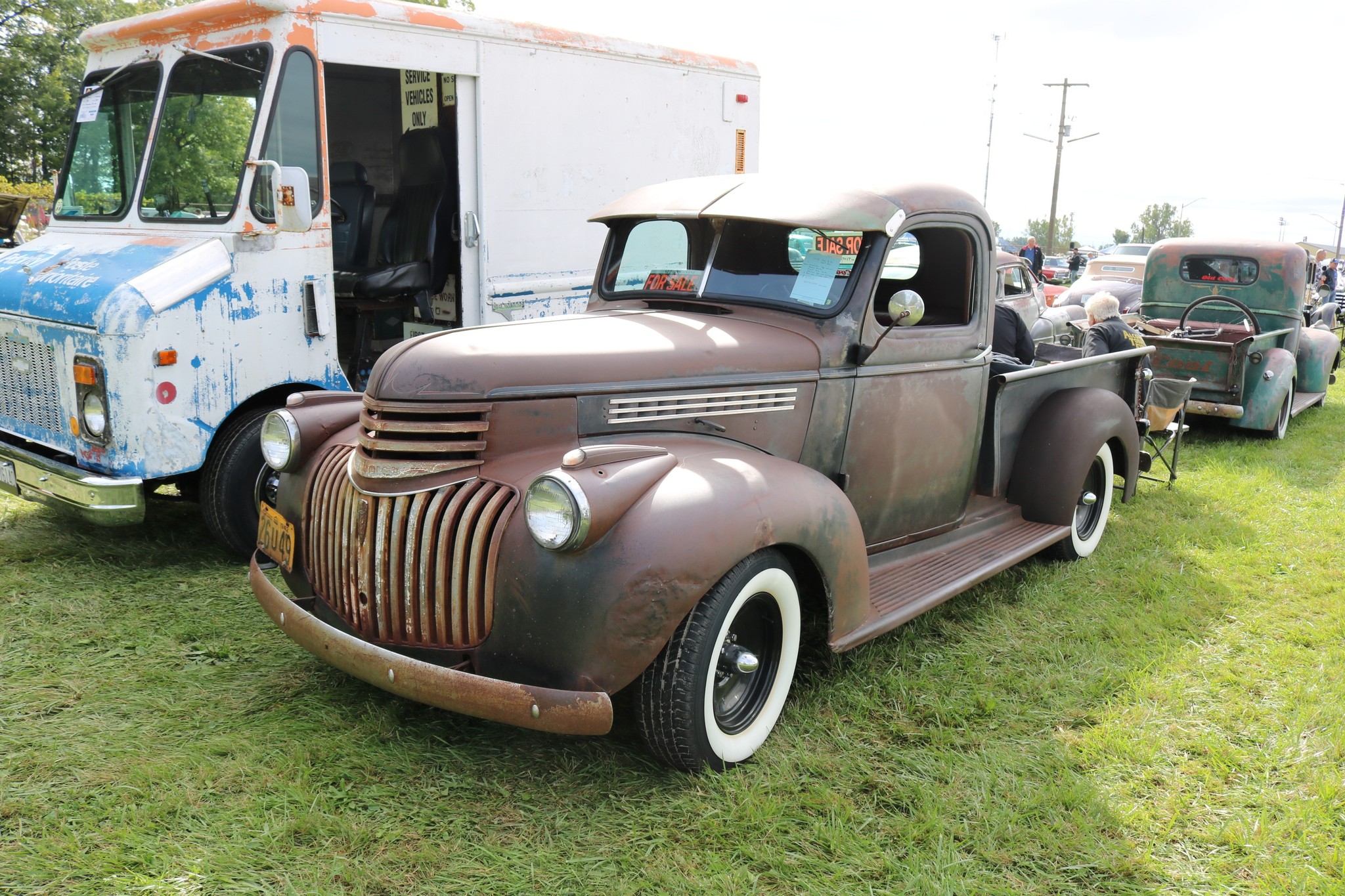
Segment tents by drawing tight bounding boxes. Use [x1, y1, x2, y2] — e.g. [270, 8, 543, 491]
[995, 237, 1020, 252]
[1068, 244, 1119, 256]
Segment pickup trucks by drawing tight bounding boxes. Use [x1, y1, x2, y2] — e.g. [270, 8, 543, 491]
[249, 177, 1173, 778]
[1066, 238, 1340, 441]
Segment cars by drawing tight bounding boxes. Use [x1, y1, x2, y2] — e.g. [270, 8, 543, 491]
[789, 234, 1152, 358]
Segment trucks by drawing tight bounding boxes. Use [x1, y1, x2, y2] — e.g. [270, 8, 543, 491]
[0, 1, 760, 567]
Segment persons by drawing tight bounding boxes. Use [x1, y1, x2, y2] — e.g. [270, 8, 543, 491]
[1087, 252, 1094, 262]
[1019, 236, 1045, 281]
[1319, 258, 1339, 332]
[1047, 291, 1154, 418]
[194, 208, 205, 218]
[1311, 249, 1327, 304]
[991, 302, 1035, 365]
[1067, 247, 1082, 284]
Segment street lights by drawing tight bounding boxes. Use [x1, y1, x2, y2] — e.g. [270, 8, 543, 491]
[1177, 197, 1208, 238]
[1308, 213, 1342, 260]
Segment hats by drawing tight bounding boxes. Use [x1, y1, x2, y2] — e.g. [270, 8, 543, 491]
[1330, 258, 1339, 263]
[1074, 248, 1078, 251]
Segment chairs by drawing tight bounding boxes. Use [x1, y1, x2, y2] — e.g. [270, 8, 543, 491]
[333, 185, 449, 391]
[330, 161, 376, 272]
[1140, 377, 1197, 492]
[1029, 342, 1082, 366]
